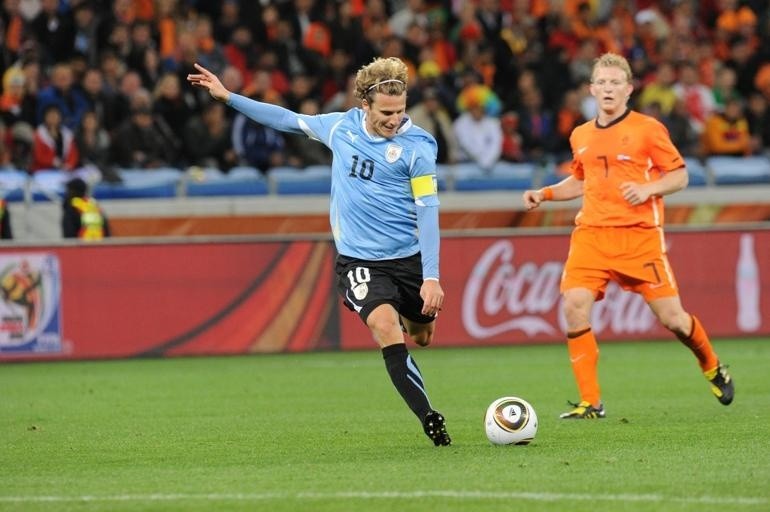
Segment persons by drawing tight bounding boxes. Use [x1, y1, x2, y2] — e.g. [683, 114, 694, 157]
[522, 52, 735, 420]
[186, 56, 452, 446]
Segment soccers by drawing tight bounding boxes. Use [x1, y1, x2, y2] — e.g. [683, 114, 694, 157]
[484, 396, 538, 444]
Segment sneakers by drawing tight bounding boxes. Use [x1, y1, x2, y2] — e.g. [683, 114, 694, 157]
[703, 359, 735, 406]
[423, 411, 451, 446]
[559, 401, 606, 419]
